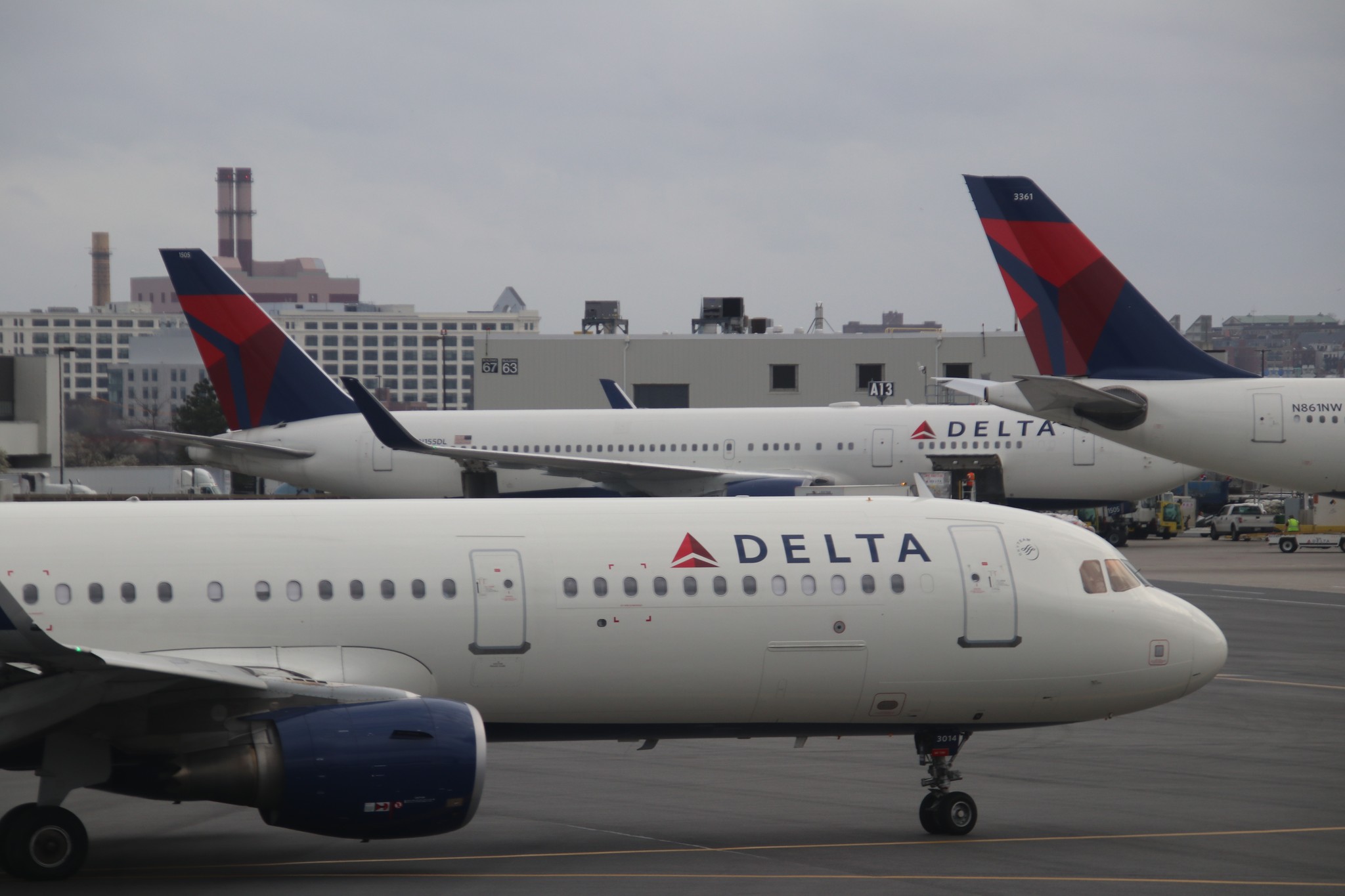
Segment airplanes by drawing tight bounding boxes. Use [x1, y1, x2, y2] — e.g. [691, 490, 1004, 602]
[0, 431, 1228, 893]
[135, 248, 1211, 548]
[927, 174, 1345, 555]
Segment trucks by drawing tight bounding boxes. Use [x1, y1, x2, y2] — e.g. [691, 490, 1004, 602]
[1063, 491, 1185, 548]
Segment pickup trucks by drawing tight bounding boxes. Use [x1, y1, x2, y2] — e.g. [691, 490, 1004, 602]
[1209, 503, 1277, 542]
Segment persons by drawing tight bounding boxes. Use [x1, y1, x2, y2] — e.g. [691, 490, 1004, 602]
[964, 470, 975, 499]
[1285, 516, 1300, 532]
